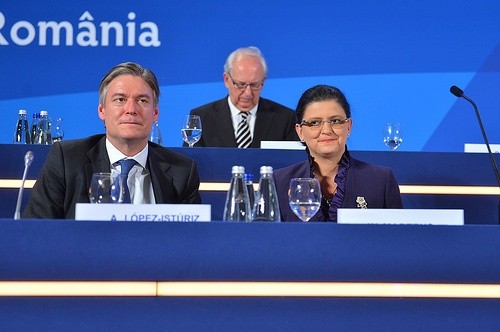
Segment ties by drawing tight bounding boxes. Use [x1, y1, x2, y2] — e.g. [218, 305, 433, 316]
[236, 111, 253, 148]
[110, 159, 137, 204]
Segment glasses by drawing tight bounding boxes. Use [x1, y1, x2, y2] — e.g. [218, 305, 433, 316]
[300, 115, 351, 129]
[228, 73, 264, 90]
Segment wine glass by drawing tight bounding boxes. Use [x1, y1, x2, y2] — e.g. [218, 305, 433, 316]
[180, 116, 202, 148]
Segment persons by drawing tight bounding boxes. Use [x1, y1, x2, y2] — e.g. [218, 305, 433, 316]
[182, 47, 300, 148]
[22, 62, 202, 220]
[273, 84, 403, 223]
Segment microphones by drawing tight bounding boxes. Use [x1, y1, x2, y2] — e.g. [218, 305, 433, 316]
[449, 85, 500, 185]
[15, 150, 35, 221]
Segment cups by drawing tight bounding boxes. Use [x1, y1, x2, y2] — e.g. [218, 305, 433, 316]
[51, 116, 65, 144]
[89, 172, 126, 203]
[383, 123, 403, 150]
[288, 177, 322, 221]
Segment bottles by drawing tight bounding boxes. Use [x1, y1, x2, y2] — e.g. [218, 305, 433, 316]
[35, 110, 52, 145]
[223, 164, 252, 222]
[150, 120, 162, 146]
[12, 110, 31, 144]
[243, 173, 255, 213]
[30, 113, 41, 144]
[251, 166, 282, 222]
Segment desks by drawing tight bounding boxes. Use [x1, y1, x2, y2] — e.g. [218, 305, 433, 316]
[0, 142, 500, 225]
[0, 219, 500, 331]
